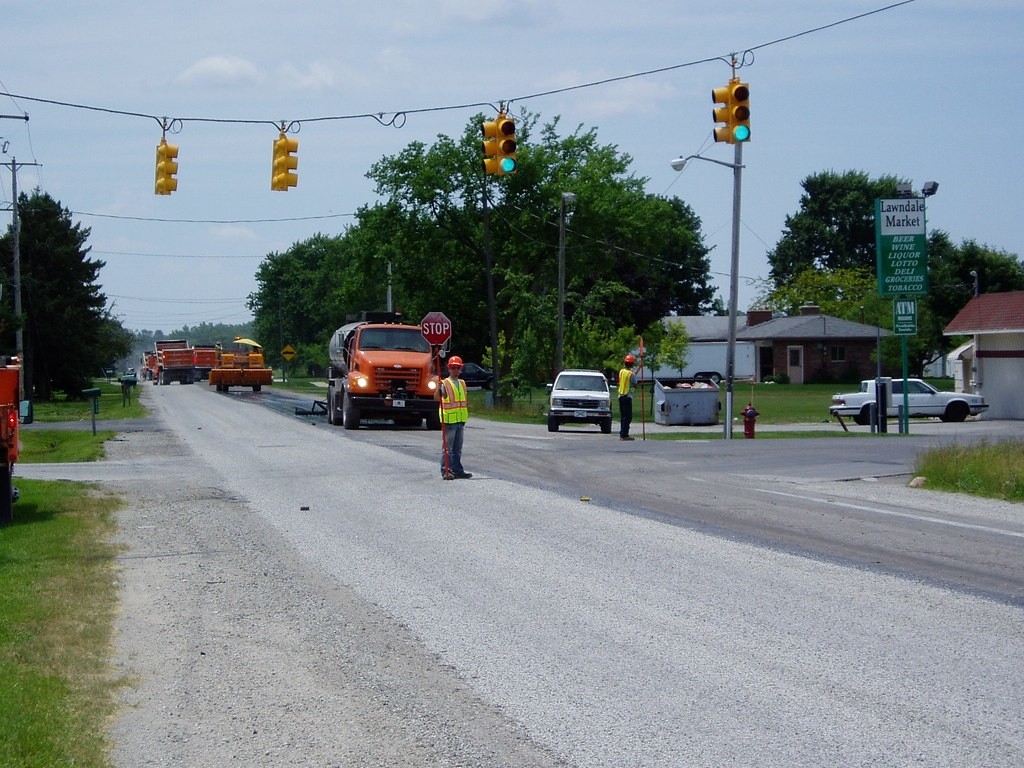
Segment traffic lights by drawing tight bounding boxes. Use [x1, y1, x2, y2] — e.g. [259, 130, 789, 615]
[495, 118, 517, 174]
[158, 143, 179, 193]
[727, 81, 752, 144]
[274, 135, 299, 190]
[712, 85, 732, 144]
[480, 121, 501, 176]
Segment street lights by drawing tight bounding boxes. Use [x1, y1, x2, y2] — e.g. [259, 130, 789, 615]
[667, 148, 744, 436]
[890, 179, 941, 433]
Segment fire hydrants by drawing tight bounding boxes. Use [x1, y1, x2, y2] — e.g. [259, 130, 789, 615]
[740, 402, 761, 438]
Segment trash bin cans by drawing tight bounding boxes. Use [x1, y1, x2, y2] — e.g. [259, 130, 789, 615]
[654, 378, 722, 426]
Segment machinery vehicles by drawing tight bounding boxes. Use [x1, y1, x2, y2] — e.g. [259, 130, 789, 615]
[209, 337, 273, 394]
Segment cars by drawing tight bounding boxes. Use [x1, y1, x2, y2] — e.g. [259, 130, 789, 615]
[441, 362, 500, 391]
[828, 378, 991, 425]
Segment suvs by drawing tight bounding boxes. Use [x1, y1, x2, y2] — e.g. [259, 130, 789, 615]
[545, 368, 615, 435]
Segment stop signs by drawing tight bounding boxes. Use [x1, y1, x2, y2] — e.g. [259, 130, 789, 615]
[421, 312, 453, 346]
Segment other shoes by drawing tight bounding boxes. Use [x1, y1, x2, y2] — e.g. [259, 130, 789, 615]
[442, 473, 454, 481]
[454, 472, 473, 479]
[618, 436, 636, 441]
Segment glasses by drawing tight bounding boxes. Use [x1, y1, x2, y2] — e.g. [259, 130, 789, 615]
[450, 366, 461, 369]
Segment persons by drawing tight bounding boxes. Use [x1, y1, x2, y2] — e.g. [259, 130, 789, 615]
[246, 346, 259, 356]
[618, 355, 642, 441]
[216, 342, 222, 367]
[434, 357, 472, 479]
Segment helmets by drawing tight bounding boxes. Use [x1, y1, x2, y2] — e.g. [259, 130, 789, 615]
[446, 356, 464, 365]
[624, 355, 636, 364]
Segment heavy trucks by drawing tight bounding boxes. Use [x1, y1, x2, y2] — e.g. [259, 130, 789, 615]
[325, 310, 443, 432]
[140, 339, 221, 385]
